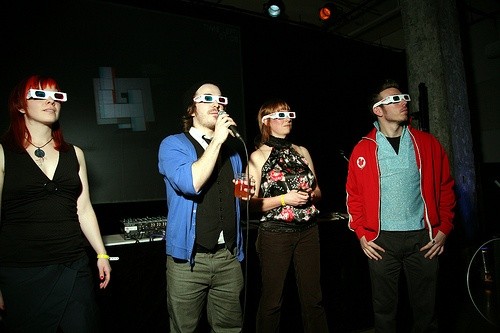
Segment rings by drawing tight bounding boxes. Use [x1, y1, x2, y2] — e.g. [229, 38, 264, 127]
[432, 239, 436, 245]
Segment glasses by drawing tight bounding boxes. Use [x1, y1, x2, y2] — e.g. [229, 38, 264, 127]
[193, 94, 228, 106]
[261, 111, 296, 123]
[372, 94, 411, 109]
[27, 88, 67, 102]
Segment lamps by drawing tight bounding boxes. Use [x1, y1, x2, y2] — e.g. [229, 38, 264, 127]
[263, 0, 286, 19]
[318, 4, 343, 23]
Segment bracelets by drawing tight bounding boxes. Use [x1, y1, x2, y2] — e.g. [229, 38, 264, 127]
[281, 194, 286, 206]
[97, 254, 109, 259]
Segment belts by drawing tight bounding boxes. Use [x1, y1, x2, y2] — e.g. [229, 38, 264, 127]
[193, 244, 227, 253]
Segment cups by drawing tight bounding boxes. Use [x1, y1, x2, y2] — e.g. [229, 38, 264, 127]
[234, 173, 253, 198]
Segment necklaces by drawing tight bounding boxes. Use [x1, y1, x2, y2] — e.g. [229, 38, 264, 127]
[25, 137, 53, 157]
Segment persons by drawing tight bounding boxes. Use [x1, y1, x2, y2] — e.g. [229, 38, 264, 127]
[245, 100, 328, 333]
[158, 83, 255, 333]
[0, 75, 112, 333]
[346, 81, 457, 333]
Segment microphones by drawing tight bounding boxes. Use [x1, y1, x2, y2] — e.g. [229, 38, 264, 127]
[218, 110, 241, 139]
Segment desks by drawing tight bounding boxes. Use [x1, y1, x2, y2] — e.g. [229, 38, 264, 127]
[86, 211, 375, 333]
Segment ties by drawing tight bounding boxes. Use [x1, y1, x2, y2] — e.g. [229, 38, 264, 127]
[202, 135, 212, 145]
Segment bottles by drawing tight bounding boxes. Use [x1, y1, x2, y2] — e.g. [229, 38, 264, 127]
[478, 239, 496, 284]
